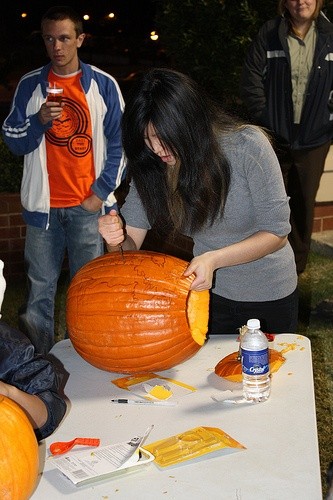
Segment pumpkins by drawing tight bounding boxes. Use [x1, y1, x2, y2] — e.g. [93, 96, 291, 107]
[0, 393, 39, 500]
[214, 349, 286, 382]
[65, 248, 210, 375]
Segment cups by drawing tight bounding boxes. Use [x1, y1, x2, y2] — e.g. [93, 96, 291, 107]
[46, 86, 63, 107]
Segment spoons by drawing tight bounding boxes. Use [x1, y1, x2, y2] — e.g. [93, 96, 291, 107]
[50, 438, 100, 454]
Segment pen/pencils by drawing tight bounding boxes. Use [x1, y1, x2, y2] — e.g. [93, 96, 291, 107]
[111, 398, 145, 405]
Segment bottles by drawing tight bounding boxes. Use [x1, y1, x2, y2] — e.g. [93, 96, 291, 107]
[239, 318, 270, 403]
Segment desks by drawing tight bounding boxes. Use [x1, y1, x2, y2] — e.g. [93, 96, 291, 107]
[29, 334, 323, 500]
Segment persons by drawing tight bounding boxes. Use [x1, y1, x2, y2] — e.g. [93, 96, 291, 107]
[238, 1, 333, 276]
[0, 298, 65, 499]
[96, 66, 299, 334]
[2, 9, 128, 356]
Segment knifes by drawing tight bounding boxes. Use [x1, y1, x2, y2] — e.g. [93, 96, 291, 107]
[117, 243, 127, 263]
